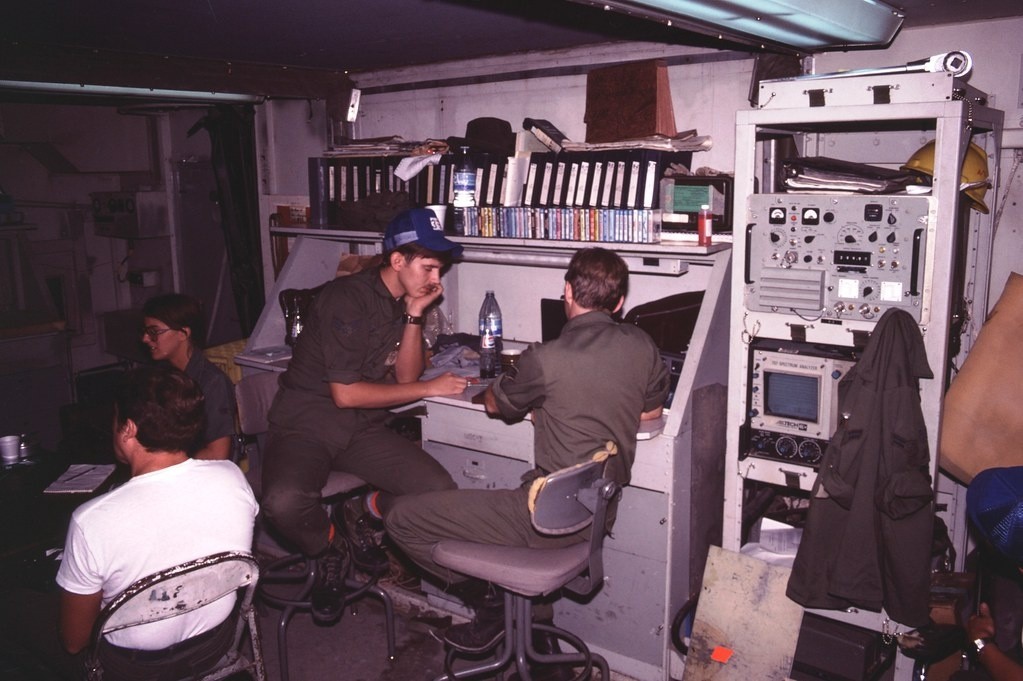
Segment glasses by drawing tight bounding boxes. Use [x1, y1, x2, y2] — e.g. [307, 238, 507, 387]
[139, 326, 172, 336]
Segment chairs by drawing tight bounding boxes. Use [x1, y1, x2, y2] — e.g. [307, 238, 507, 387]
[236, 370, 397, 681]
[433, 450, 624, 681]
[82, 551, 267, 681]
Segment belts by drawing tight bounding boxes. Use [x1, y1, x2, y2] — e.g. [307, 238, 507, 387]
[100, 624, 226, 661]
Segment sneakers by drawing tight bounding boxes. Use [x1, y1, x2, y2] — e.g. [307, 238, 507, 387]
[329, 497, 391, 576]
[443, 607, 513, 656]
[310, 530, 349, 625]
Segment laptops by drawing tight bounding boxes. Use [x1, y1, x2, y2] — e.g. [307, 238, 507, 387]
[539, 298, 568, 344]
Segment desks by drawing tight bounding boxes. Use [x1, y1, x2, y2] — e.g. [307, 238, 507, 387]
[0, 417, 139, 557]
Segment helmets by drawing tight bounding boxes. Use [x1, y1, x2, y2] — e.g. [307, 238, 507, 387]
[899, 134, 991, 215]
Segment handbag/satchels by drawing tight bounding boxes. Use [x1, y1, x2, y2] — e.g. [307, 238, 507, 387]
[411, 138, 451, 156]
[278, 281, 333, 348]
[583, 59, 699, 144]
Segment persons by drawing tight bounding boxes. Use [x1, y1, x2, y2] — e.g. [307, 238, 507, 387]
[142, 294, 237, 459]
[384, 249, 669, 655]
[950, 601, 1023, 681]
[1, 368, 262, 681]
[261, 209, 469, 627]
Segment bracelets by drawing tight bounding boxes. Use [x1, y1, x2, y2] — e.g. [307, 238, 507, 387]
[968, 637, 993, 664]
[402, 313, 423, 323]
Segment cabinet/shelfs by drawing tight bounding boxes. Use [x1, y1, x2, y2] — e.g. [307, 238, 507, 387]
[723, 99, 1004, 562]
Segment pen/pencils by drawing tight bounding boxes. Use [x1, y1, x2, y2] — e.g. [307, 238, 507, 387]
[63, 465, 98, 483]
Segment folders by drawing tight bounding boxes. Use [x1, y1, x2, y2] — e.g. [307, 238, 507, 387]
[523, 118, 572, 156]
[326, 161, 456, 207]
[521, 152, 694, 209]
[475, 164, 512, 204]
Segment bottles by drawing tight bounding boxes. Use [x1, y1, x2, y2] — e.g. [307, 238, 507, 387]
[453, 146, 477, 209]
[478, 290, 503, 338]
[480, 317, 496, 379]
[291, 302, 304, 344]
[698, 204, 712, 247]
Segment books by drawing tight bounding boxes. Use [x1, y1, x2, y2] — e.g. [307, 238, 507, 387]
[42, 463, 116, 493]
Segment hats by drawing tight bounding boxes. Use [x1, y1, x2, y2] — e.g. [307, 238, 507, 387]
[445, 117, 517, 157]
[381, 207, 464, 258]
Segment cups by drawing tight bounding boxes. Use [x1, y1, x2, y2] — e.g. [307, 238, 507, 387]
[0, 435, 21, 466]
[500, 349, 521, 372]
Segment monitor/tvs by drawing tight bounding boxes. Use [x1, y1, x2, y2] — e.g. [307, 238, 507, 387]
[764, 371, 817, 423]
